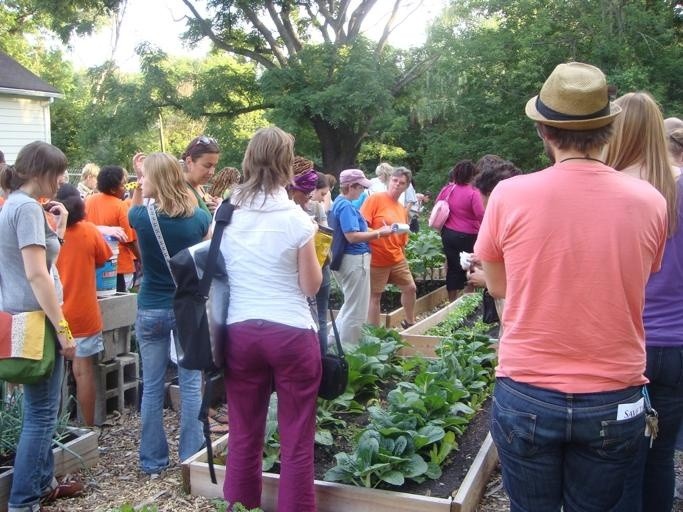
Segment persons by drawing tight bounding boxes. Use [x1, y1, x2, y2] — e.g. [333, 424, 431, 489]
[473, 62, 669, 512]
[180, 137, 242, 217]
[0, 141, 85, 512]
[663, 117, 682, 168]
[286, 159, 433, 354]
[435, 155, 523, 326]
[127, 152, 216, 474]
[602, 93, 683, 512]
[1, 162, 141, 429]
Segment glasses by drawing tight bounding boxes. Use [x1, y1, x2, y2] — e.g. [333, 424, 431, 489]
[189, 135, 217, 150]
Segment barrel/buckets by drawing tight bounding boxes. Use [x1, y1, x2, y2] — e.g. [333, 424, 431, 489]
[95, 236, 120, 297]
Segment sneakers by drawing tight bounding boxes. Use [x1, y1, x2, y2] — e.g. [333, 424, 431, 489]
[40, 482, 84, 504]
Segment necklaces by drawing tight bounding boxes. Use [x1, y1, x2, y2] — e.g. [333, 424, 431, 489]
[560, 153, 604, 163]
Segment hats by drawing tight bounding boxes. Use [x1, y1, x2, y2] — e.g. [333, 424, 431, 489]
[525, 62, 622, 130]
[340, 168, 371, 189]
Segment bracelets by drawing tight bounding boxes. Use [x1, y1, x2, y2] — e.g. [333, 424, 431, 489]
[135, 188, 142, 191]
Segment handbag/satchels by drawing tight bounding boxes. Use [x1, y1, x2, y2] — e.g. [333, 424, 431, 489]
[318, 352, 350, 400]
[170, 238, 229, 370]
[0, 309, 56, 384]
[326, 210, 346, 270]
[429, 201, 450, 231]
